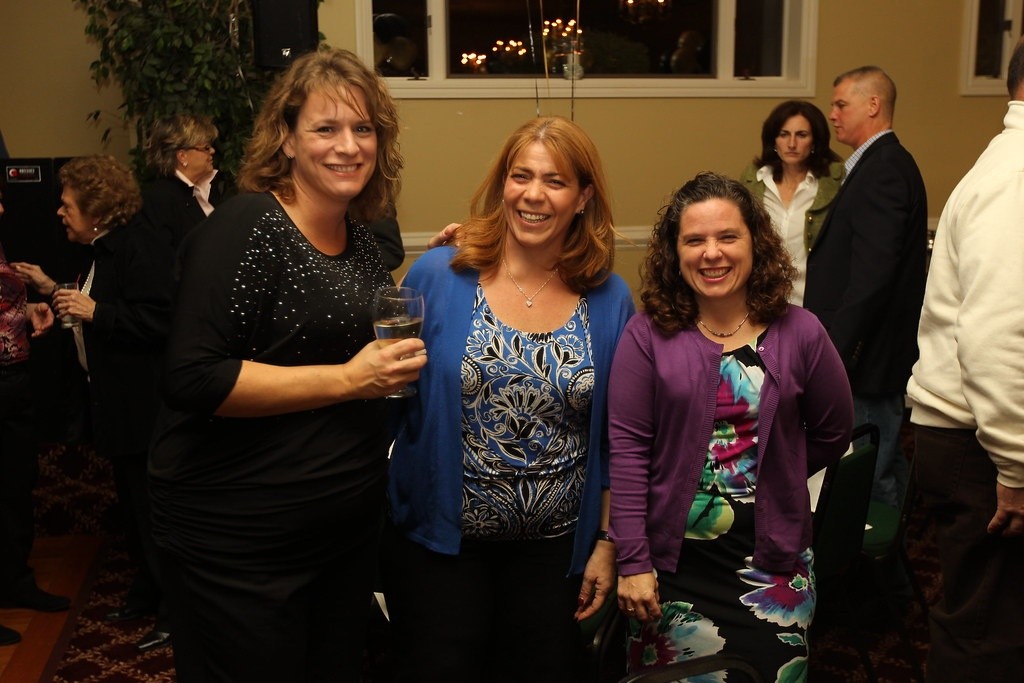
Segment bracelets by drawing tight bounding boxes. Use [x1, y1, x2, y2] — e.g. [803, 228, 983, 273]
[598, 530, 616, 544]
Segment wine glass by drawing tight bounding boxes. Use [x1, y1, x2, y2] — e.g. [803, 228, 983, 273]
[371, 286, 425, 398]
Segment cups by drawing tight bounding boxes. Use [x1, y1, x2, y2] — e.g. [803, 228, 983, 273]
[54, 282, 81, 329]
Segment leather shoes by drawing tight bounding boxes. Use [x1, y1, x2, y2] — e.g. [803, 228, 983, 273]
[102, 602, 139, 622]
[134, 630, 174, 652]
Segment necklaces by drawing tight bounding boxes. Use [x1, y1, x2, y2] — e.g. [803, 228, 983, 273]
[502, 258, 559, 309]
[697, 310, 749, 337]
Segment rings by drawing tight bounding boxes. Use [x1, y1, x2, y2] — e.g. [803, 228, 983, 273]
[627, 607, 636, 612]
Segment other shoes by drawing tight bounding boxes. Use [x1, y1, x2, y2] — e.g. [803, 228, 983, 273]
[0, 624, 22, 645]
[0, 585, 71, 613]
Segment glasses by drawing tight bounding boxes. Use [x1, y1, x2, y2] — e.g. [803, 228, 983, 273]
[175, 142, 211, 153]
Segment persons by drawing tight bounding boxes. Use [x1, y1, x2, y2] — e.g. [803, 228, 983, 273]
[158, 40, 476, 683]
[0, 131, 79, 644]
[8, 115, 242, 637]
[394, 112, 634, 683]
[607, 170, 860, 683]
[736, 35, 1024, 683]
